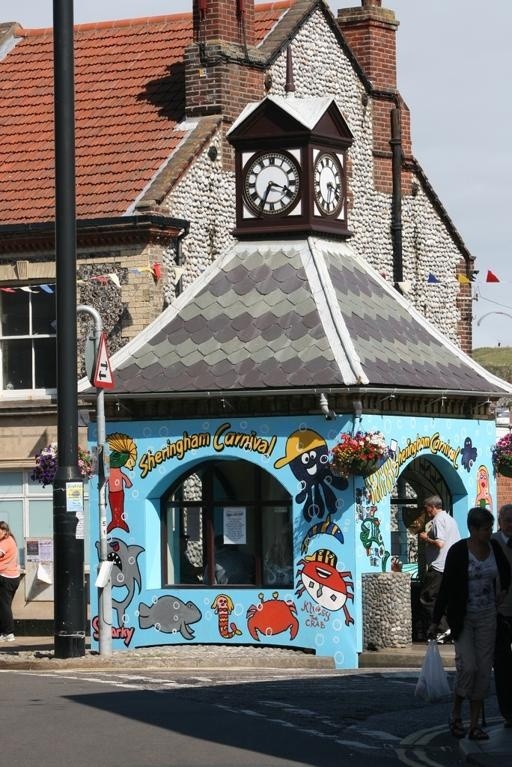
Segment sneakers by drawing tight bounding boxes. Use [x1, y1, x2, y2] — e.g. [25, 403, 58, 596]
[428, 627, 451, 644]
[0, 633, 15, 642]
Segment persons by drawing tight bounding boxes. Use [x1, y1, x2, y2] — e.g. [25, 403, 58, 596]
[1, 520, 27, 644]
[490, 502, 512, 731]
[418, 493, 463, 646]
[427, 507, 511, 742]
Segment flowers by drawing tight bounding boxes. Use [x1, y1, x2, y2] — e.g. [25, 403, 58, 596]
[491, 432, 512, 477]
[30, 442, 98, 488]
[327, 430, 389, 478]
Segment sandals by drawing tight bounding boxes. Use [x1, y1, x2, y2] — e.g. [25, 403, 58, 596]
[449, 714, 490, 740]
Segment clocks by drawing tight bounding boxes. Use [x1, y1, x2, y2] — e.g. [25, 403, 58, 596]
[239, 147, 302, 218]
[312, 148, 345, 219]
